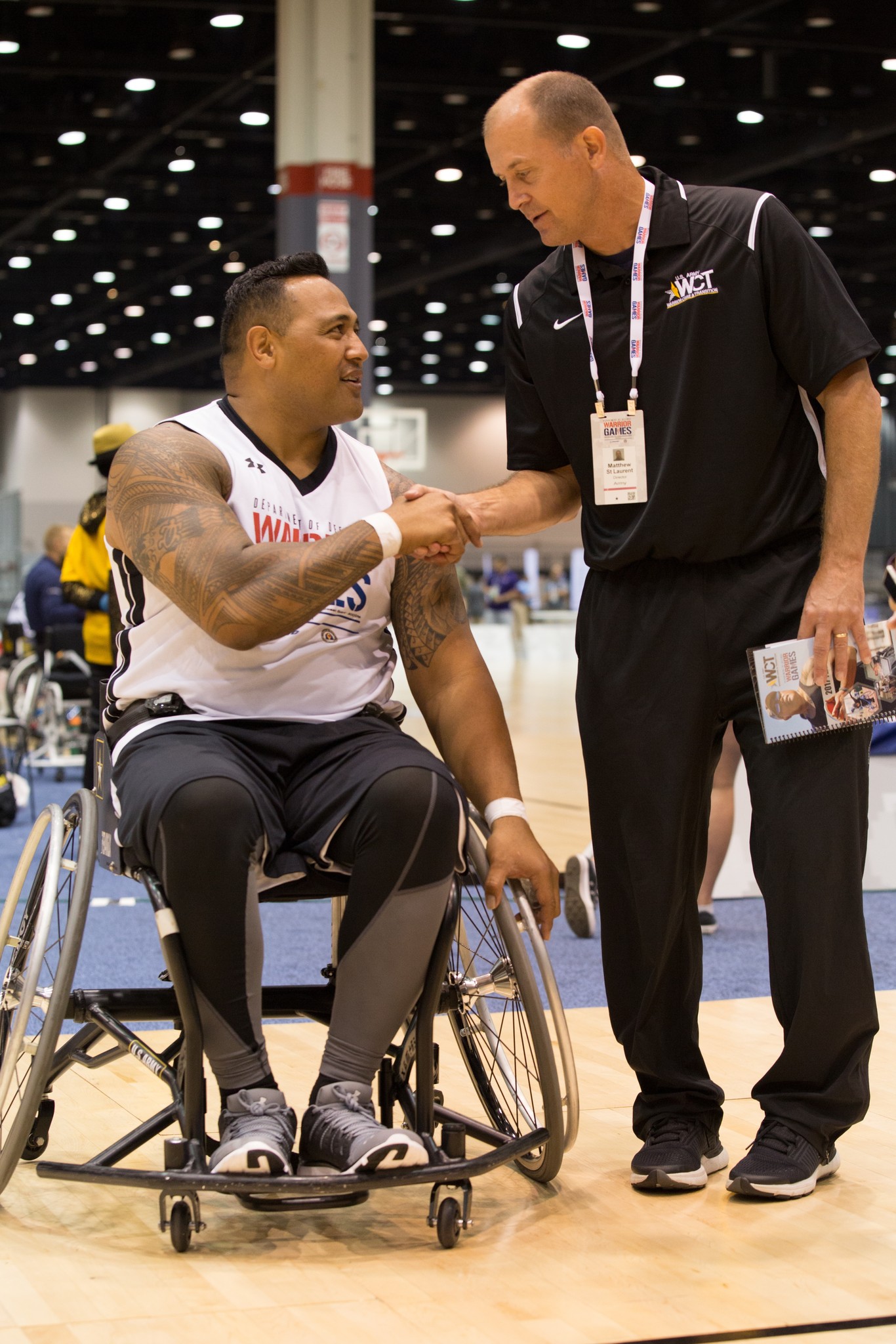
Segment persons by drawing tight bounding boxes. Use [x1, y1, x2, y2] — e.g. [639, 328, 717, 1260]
[103, 253, 562, 1174]
[0, 420, 135, 791]
[404, 68, 896, 1196]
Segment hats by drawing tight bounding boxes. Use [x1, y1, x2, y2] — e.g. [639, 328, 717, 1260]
[88, 422, 137, 465]
[764, 691, 781, 720]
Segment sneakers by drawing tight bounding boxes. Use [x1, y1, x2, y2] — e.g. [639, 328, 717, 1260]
[630, 1113, 730, 1194]
[724, 1112, 840, 1204]
[298, 1082, 431, 1175]
[209, 1085, 298, 1176]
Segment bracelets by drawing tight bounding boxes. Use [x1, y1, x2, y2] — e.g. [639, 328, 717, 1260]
[360, 508, 402, 561]
[484, 795, 529, 833]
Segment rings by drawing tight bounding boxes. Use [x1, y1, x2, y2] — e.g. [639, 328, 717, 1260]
[835, 632, 847, 639]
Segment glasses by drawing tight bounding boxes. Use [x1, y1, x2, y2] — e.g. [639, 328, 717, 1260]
[770, 690, 780, 715]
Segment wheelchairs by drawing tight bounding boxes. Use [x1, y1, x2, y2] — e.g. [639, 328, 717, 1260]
[2, 721, 582, 1257]
[0, 649, 117, 828]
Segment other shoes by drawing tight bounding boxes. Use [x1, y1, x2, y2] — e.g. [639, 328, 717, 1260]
[698, 909, 720, 936]
[565, 852, 599, 939]
